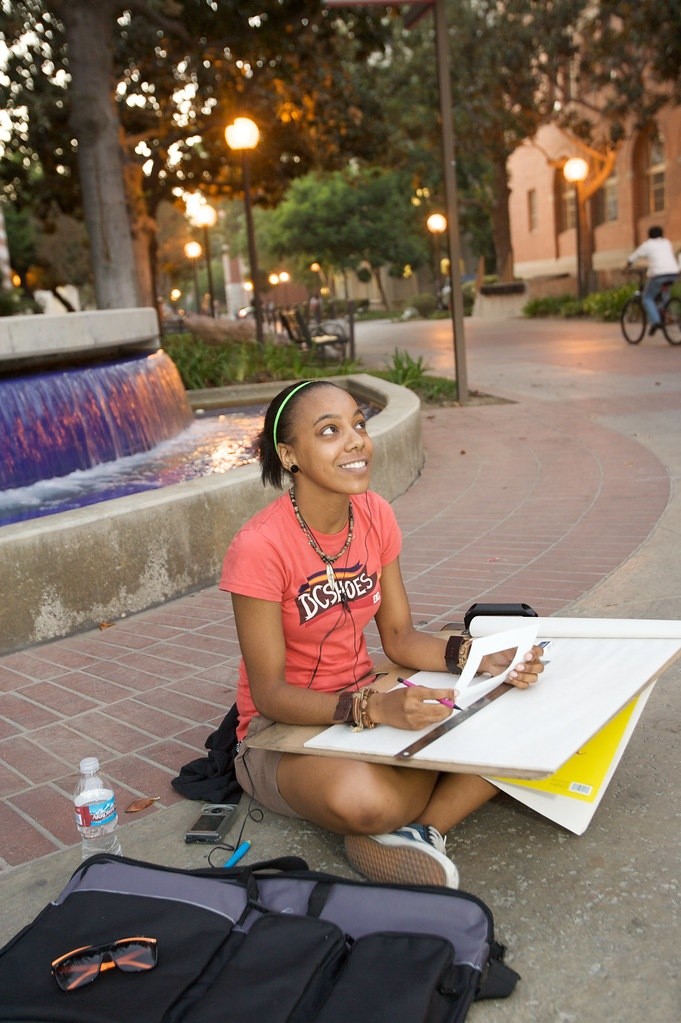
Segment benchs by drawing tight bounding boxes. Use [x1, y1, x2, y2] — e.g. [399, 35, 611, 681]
[279, 307, 349, 368]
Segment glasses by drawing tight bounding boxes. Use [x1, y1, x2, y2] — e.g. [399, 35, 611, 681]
[51, 937, 158, 991]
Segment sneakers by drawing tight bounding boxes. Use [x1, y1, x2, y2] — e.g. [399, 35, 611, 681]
[346, 822, 459, 891]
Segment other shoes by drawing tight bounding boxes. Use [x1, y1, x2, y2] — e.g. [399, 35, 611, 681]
[649, 323, 662, 336]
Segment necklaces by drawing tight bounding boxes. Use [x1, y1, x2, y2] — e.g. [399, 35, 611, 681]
[288, 487, 354, 589]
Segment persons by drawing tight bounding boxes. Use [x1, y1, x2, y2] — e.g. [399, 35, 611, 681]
[218, 378, 546, 890]
[623, 225, 680, 337]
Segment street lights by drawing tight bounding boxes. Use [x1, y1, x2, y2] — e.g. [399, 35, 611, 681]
[190, 203, 218, 320]
[185, 241, 204, 315]
[563, 157, 590, 301]
[224, 116, 260, 341]
[427, 214, 448, 312]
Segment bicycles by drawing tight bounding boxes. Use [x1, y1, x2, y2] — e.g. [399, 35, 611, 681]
[620, 266, 681, 347]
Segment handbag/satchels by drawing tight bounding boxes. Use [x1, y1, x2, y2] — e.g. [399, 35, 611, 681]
[0, 853, 520, 1023]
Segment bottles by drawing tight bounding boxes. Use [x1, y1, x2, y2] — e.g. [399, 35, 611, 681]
[72, 757, 123, 864]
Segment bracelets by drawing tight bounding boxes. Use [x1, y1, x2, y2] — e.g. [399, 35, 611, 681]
[459, 638, 483, 677]
[352, 685, 380, 734]
[333, 690, 357, 727]
[445, 634, 467, 675]
